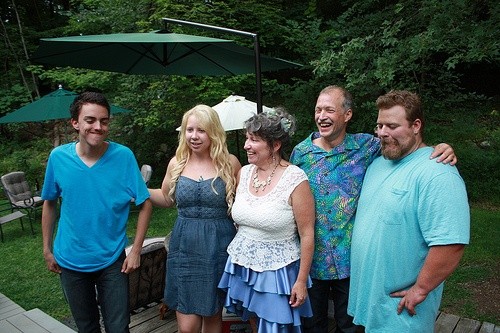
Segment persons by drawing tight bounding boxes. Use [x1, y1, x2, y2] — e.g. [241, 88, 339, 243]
[217, 112, 314, 332]
[41, 93, 151, 332]
[148, 104, 241, 332]
[288, 85, 457, 333]
[348, 88, 470, 333]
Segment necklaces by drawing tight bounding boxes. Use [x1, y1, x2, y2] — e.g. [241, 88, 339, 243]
[252, 158, 282, 192]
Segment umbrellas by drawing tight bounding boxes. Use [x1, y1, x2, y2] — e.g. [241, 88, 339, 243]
[38, 14, 304, 165]
[1, 84, 135, 122]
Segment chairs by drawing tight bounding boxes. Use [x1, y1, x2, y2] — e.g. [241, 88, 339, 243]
[1, 171, 52, 236]
[125, 237, 167, 312]
[0, 202, 27, 243]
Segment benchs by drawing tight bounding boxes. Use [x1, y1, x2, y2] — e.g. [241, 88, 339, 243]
[128, 164, 152, 212]
